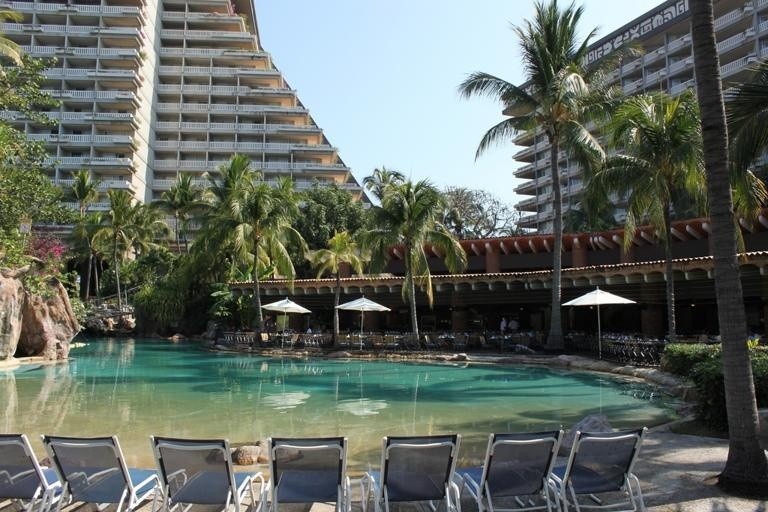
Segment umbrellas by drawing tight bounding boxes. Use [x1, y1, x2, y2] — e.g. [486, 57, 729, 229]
[258, 296, 313, 349]
[332, 294, 393, 333]
[562, 285, 639, 361]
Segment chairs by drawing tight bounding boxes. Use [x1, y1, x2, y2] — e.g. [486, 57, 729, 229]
[0, 434, 62, 512]
[40, 434, 163, 512]
[360, 434, 461, 512]
[453, 429, 565, 512]
[551, 426, 649, 512]
[151, 436, 263, 512]
[262, 436, 352, 512]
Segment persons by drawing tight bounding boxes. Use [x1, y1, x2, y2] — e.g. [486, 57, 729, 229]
[222, 318, 764, 351]
